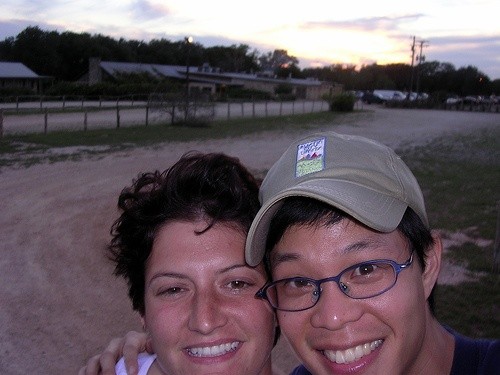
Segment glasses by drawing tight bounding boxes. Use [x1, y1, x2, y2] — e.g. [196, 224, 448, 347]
[254, 250, 415, 312]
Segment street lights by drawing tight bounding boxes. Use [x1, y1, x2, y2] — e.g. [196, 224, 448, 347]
[184, 35, 193, 116]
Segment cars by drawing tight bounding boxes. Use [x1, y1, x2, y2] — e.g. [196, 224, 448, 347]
[348, 86, 500, 107]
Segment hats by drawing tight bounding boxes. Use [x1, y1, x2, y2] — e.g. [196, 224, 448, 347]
[245, 132, 429, 267]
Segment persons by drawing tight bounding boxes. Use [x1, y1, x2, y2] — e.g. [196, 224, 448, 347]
[78, 132, 500, 375]
[98, 151, 281, 375]
